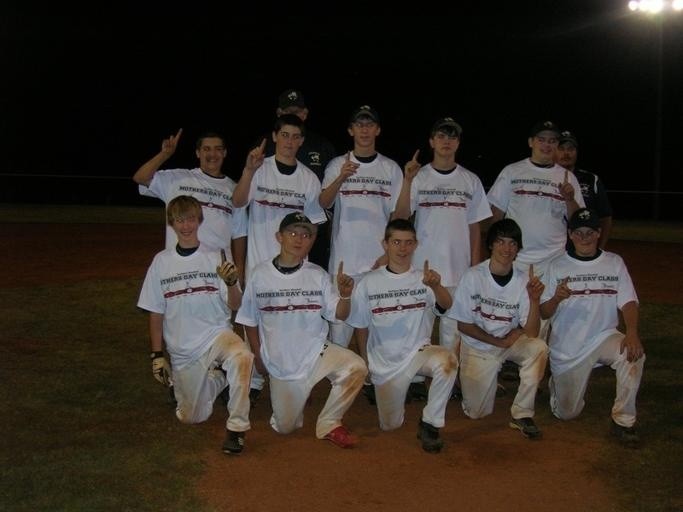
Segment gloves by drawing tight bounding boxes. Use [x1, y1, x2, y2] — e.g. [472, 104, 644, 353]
[216, 248, 239, 287]
[148, 351, 173, 388]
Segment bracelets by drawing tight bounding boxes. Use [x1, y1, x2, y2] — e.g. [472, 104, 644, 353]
[148, 352, 165, 359]
[225, 279, 237, 287]
[338, 293, 351, 300]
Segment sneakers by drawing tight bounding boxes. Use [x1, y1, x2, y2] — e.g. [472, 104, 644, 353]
[222, 425, 245, 454]
[501, 360, 519, 381]
[611, 414, 641, 448]
[417, 416, 443, 454]
[509, 413, 541, 438]
[409, 378, 429, 403]
[362, 382, 376, 405]
[316, 422, 359, 448]
[213, 365, 230, 407]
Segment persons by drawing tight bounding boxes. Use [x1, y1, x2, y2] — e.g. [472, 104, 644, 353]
[449, 218, 548, 440]
[395, 118, 493, 403]
[137, 195, 255, 453]
[352, 219, 460, 454]
[236, 212, 367, 447]
[231, 115, 331, 406]
[258, 93, 331, 178]
[319, 105, 403, 405]
[558, 128, 613, 251]
[486, 120, 586, 397]
[540, 209, 646, 450]
[133, 127, 247, 411]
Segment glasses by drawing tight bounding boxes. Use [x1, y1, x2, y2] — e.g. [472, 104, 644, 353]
[281, 108, 303, 116]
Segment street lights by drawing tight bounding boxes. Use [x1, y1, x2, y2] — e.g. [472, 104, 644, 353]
[627, 0, 682, 216]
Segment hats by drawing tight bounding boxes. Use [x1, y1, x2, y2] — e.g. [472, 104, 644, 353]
[532, 120, 559, 139]
[279, 91, 306, 108]
[351, 105, 379, 123]
[279, 212, 318, 235]
[559, 128, 576, 147]
[433, 117, 462, 142]
[569, 208, 599, 236]
